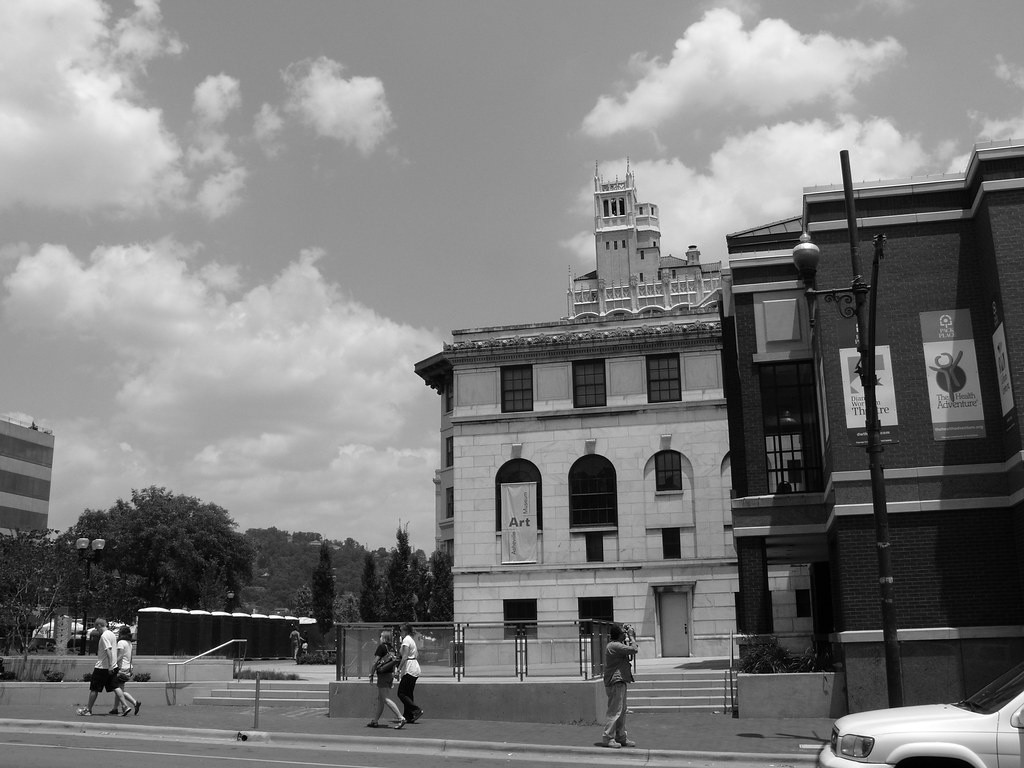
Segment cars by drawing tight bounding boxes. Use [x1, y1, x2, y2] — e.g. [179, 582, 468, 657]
[816, 658, 1024, 768]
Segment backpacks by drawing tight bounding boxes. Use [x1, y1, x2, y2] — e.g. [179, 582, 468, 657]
[290, 634, 299, 646]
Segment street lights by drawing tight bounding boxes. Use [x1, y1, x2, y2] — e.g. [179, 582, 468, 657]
[76, 536, 106, 653]
[792, 228, 905, 707]
[227, 590, 234, 612]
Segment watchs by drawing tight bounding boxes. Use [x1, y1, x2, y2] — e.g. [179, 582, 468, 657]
[369, 674, 373, 677]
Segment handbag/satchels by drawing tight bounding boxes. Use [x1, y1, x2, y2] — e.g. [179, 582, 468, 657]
[374, 644, 400, 673]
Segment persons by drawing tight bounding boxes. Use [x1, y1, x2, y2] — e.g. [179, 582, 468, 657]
[366, 622, 424, 729]
[77, 619, 141, 717]
[290, 626, 304, 660]
[601, 625, 638, 747]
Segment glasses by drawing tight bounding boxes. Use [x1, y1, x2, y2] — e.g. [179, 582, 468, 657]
[380, 636, 381, 637]
[399, 628, 401, 632]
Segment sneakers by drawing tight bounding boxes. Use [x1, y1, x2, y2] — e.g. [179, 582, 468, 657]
[134, 701, 141, 715]
[367, 720, 378, 728]
[76, 708, 92, 716]
[601, 739, 621, 748]
[615, 738, 635, 747]
[109, 709, 118, 714]
[411, 706, 423, 721]
[394, 718, 407, 729]
[121, 706, 131, 716]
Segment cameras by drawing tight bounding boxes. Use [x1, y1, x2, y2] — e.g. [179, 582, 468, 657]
[624, 624, 633, 631]
